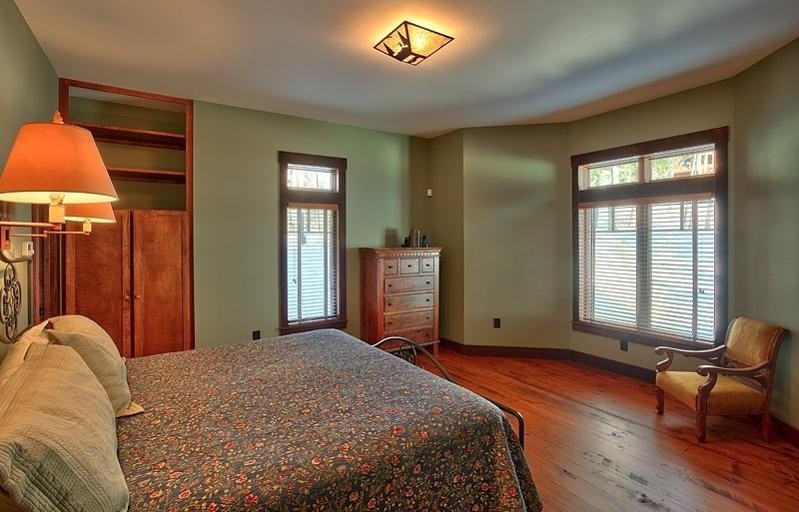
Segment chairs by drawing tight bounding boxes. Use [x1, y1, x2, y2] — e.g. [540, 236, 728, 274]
[654, 317, 790, 443]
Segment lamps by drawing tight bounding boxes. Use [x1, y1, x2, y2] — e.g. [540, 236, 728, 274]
[1, 110, 120, 251]
[373, 21, 455, 66]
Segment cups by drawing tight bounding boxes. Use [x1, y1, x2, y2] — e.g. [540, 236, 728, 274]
[410, 230, 421, 247]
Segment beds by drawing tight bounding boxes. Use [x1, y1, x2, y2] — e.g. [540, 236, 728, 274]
[1, 251, 543, 512]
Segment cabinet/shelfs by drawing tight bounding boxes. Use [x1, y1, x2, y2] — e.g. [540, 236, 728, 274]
[59, 77, 195, 358]
[358, 247, 444, 354]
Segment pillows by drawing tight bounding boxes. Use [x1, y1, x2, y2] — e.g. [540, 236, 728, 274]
[1, 318, 48, 388]
[47, 315, 145, 417]
[1, 319, 130, 512]
